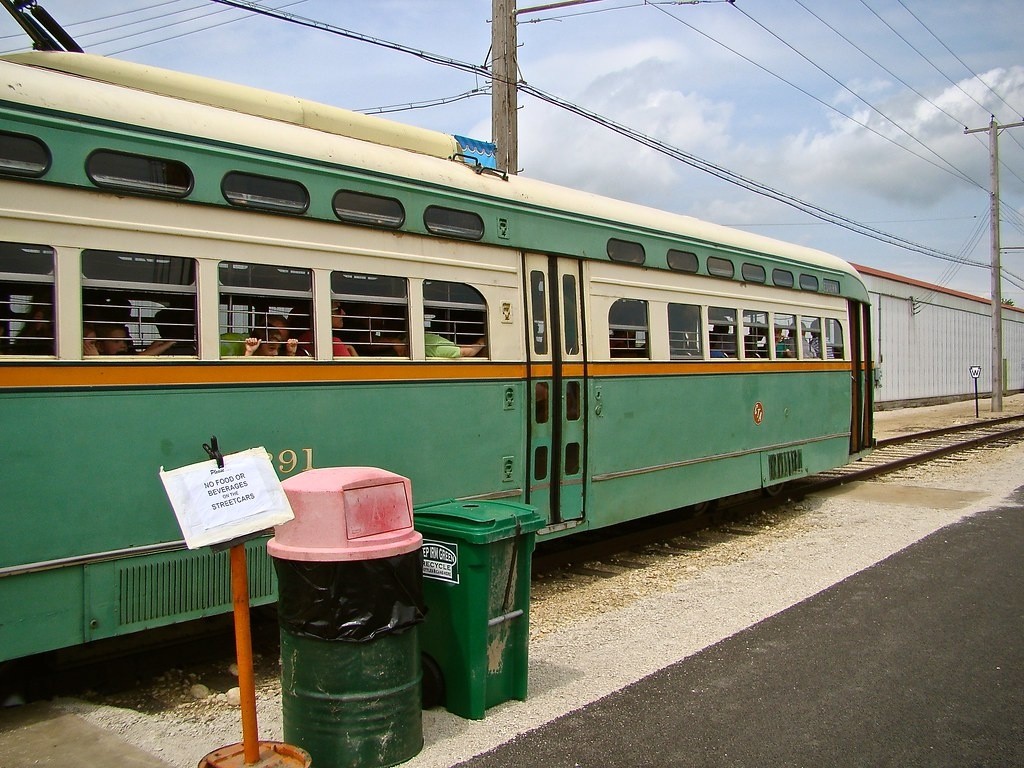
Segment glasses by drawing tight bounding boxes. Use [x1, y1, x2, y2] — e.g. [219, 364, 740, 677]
[332, 306, 342, 315]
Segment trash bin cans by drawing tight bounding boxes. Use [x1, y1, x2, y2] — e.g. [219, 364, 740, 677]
[413, 496, 547, 721]
[266, 466, 426, 768]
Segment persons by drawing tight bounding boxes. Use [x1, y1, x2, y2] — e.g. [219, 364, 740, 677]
[0, 283, 488, 357]
[534, 318, 836, 360]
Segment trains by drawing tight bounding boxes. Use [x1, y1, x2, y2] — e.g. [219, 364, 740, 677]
[1, 47, 875, 661]
